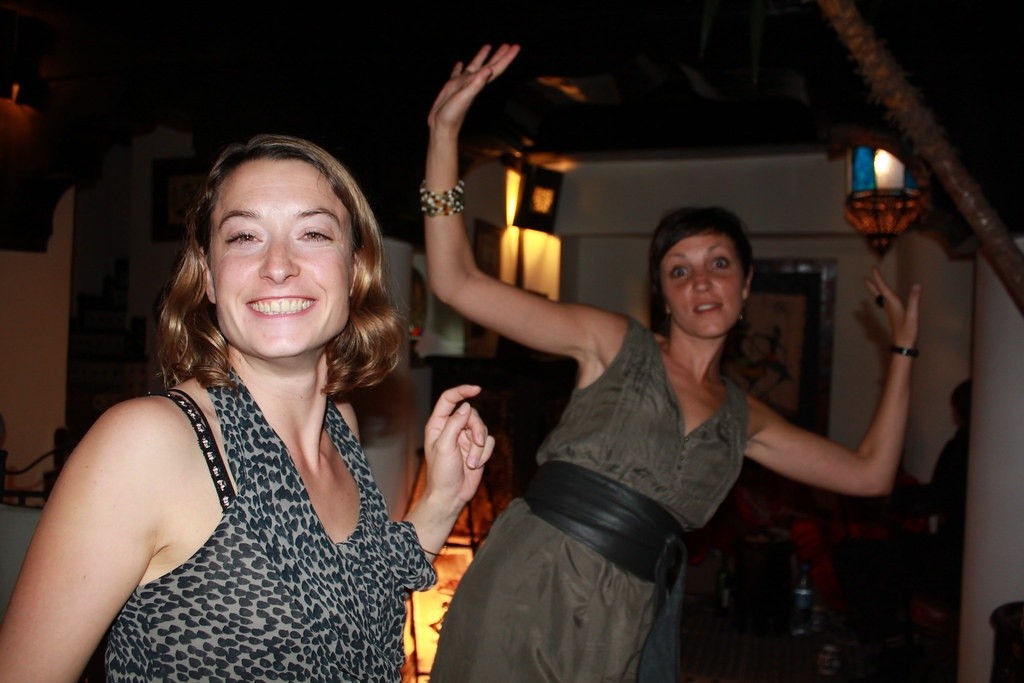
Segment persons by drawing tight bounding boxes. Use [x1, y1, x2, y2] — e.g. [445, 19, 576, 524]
[2, 134, 495, 681]
[419, 44, 923, 682]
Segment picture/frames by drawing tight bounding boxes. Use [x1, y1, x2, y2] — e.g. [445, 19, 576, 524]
[648, 256, 839, 440]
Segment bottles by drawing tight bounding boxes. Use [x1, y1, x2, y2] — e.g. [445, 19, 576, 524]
[792, 562, 819, 640]
[716, 552, 733, 610]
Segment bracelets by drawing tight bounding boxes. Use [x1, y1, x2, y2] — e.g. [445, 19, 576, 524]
[419, 180, 464, 219]
[890, 346, 919, 357]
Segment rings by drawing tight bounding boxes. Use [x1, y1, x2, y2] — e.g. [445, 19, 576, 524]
[875, 294, 886, 308]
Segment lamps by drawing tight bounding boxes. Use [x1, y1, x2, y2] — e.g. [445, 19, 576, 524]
[844, 130, 931, 256]
[395, 446, 489, 683]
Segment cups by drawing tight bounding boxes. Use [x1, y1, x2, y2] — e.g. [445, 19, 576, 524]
[817, 645, 841, 676]
